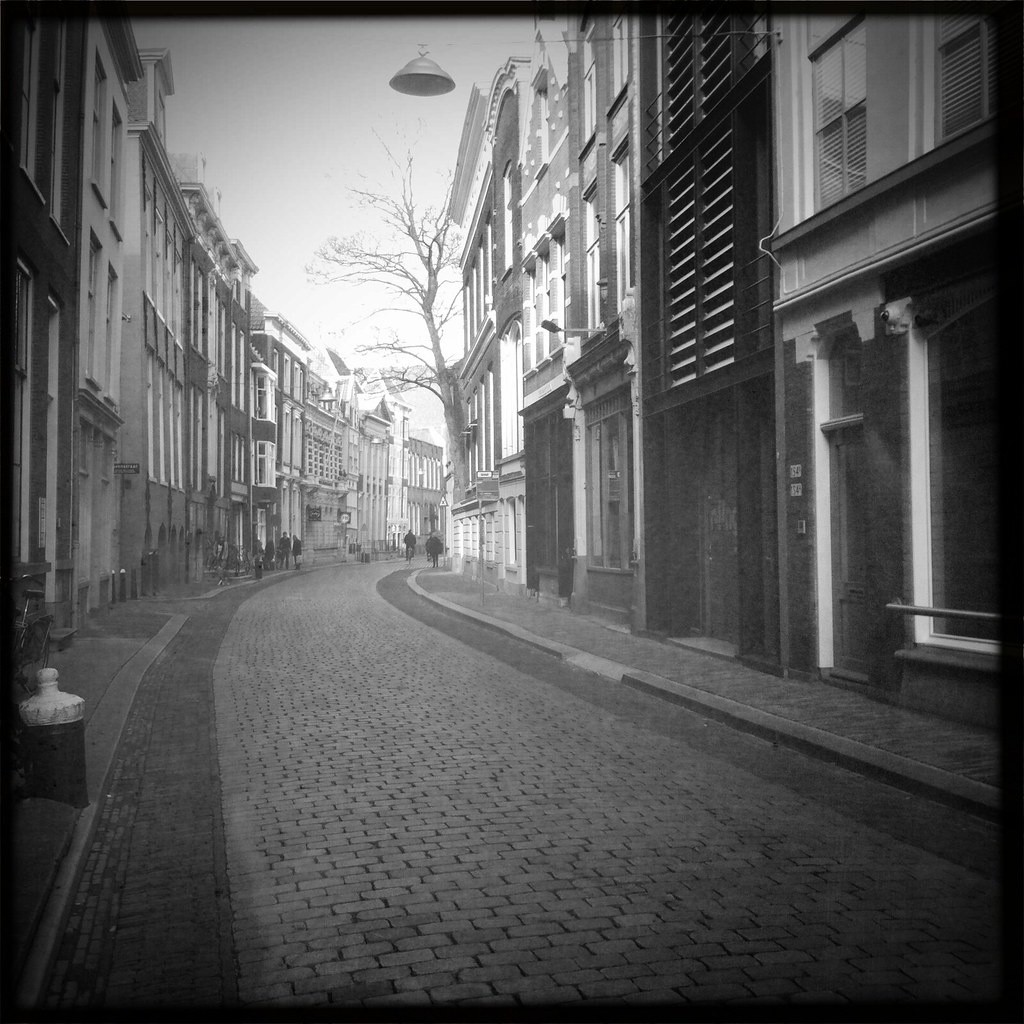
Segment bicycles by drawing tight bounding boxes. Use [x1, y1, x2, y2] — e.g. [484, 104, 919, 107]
[4, 571, 57, 701]
[406, 541, 415, 566]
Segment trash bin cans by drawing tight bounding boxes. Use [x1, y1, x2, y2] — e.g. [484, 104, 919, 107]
[140, 549, 159, 595]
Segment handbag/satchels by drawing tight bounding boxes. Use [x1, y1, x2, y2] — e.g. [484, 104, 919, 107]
[296, 554, 303, 565]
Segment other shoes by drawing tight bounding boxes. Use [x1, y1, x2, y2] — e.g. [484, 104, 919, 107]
[295, 567, 300, 570]
[279, 567, 282, 570]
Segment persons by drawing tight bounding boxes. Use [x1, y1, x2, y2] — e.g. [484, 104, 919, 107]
[404, 530, 417, 561]
[426, 532, 443, 568]
[212, 532, 302, 586]
